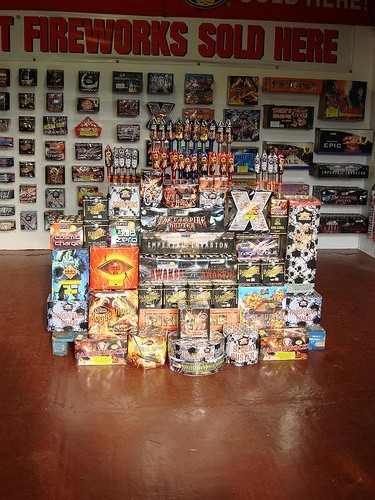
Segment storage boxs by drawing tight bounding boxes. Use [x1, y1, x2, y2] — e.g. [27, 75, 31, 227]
[0, 65, 375, 376]
